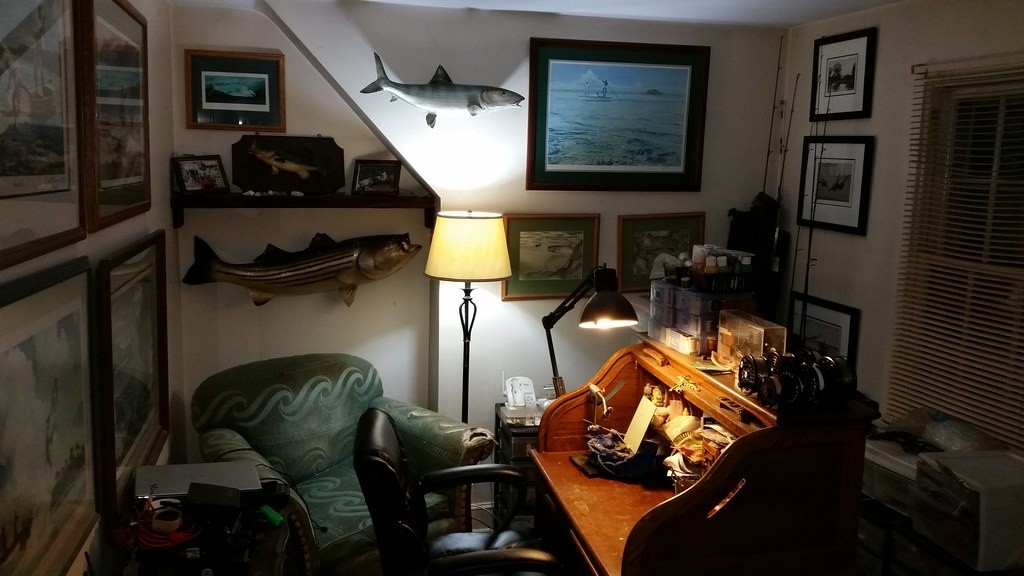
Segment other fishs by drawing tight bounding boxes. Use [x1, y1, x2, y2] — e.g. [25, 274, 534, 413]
[211, 83, 256, 99]
[253, 147, 317, 180]
[360, 52, 526, 128]
[183, 232, 423, 307]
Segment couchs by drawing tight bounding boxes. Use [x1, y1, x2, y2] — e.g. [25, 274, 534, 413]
[190, 353, 494, 576]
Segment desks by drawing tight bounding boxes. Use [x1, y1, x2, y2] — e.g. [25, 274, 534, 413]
[527, 333, 867, 575]
[97, 483, 292, 576]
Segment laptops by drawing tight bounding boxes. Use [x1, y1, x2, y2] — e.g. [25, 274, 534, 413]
[134, 461, 262, 499]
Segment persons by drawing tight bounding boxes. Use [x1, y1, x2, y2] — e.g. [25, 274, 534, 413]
[602, 80, 608, 93]
[584, 81, 591, 95]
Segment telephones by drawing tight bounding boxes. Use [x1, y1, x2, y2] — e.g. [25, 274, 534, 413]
[501, 371, 538, 410]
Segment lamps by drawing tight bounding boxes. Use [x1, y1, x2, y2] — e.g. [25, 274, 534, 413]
[425, 210, 513, 424]
[542, 263, 639, 397]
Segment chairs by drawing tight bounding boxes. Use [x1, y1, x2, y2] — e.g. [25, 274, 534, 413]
[353, 408, 558, 576]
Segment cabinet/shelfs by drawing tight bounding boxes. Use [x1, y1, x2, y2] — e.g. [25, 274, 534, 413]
[863, 440, 1024, 576]
[492, 401, 559, 534]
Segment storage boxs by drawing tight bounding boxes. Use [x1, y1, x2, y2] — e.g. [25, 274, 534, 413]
[647, 282, 742, 359]
[718, 310, 787, 369]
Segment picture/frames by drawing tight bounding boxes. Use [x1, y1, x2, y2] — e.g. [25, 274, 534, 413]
[81, 0, 151, 233]
[0, 254, 103, 576]
[787, 291, 860, 373]
[232, 132, 345, 193]
[795, 136, 875, 237]
[183, 49, 287, 133]
[94, 230, 174, 519]
[503, 213, 600, 301]
[809, 28, 878, 122]
[170, 154, 231, 196]
[350, 159, 402, 197]
[617, 211, 705, 293]
[526, 37, 711, 192]
[0, 0, 88, 270]
